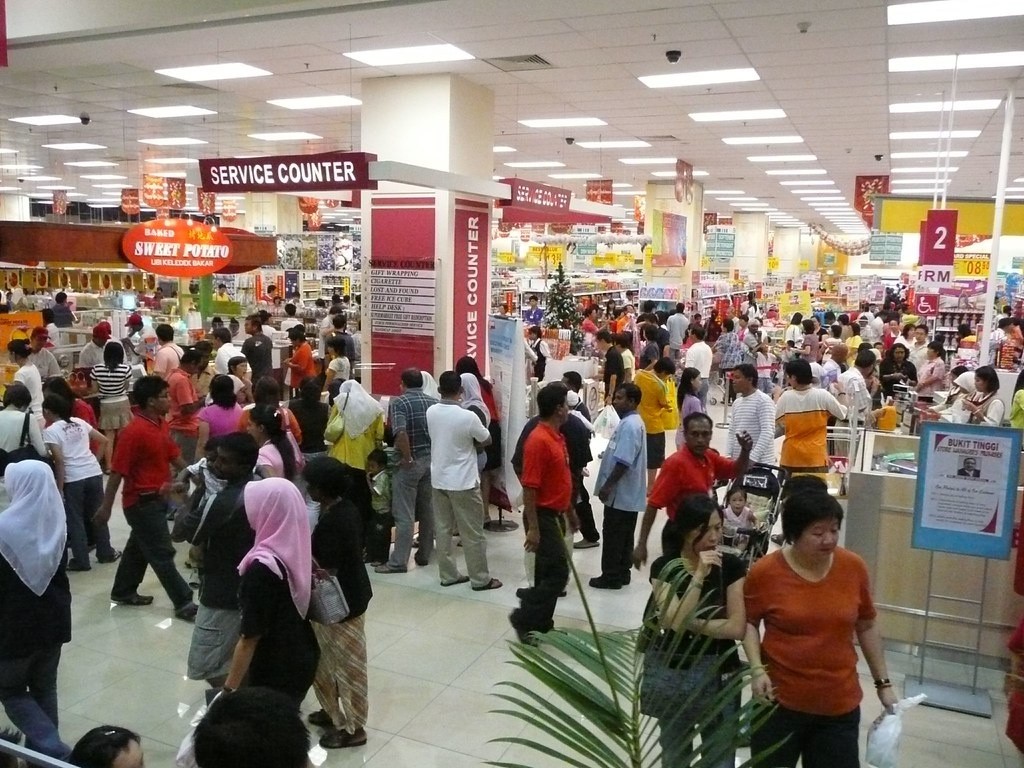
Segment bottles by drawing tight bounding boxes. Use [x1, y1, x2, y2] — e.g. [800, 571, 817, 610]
[876, 400, 897, 430]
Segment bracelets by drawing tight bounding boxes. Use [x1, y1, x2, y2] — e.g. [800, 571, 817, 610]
[403, 456, 413, 464]
[751, 665, 765, 670]
[690, 580, 702, 589]
[874, 679, 891, 688]
[224, 687, 236, 692]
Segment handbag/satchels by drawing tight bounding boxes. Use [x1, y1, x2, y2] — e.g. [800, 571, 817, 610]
[324, 411, 345, 442]
[305, 556, 349, 625]
[177, 692, 224, 768]
[593, 404, 622, 438]
[867, 694, 928, 767]
[638, 646, 726, 722]
[281, 408, 306, 469]
[660, 381, 679, 430]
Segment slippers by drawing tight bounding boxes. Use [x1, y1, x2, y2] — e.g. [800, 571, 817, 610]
[573, 539, 599, 547]
[472, 578, 503, 590]
[441, 575, 470, 586]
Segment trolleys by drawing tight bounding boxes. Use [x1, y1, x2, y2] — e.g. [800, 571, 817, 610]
[726, 352, 782, 381]
[675, 347, 725, 406]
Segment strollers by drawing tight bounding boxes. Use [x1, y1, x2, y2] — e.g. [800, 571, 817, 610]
[719, 461, 788, 575]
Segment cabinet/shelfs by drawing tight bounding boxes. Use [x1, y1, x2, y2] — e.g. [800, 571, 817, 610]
[927, 308, 997, 356]
[567, 289, 638, 310]
[303, 284, 362, 302]
[693, 288, 756, 319]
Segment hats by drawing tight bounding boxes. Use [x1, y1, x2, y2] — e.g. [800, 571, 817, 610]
[99, 322, 111, 335]
[31, 326, 51, 339]
[93, 325, 112, 339]
[567, 390, 579, 406]
[125, 314, 142, 326]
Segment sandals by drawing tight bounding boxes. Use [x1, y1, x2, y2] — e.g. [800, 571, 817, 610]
[185, 559, 204, 590]
[104, 468, 111, 474]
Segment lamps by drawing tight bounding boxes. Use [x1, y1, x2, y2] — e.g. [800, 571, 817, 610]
[178, 209, 194, 226]
[203, 215, 217, 233]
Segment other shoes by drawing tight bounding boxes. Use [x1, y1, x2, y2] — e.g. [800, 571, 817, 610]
[319, 727, 367, 748]
[508, 612, 538, 650]
[376, 564, 407, 573]
[414, 553, 428, 566]
[113, 549, 122, 560]
[590, 576, 631, 589]
[69, 558, 91, 570]
[582, 467, 589, 476]
[111, 594, 153, 605]
[175, 604, 199, 618]
[308, 710, 336, 729]
[598, 450, 605, 459]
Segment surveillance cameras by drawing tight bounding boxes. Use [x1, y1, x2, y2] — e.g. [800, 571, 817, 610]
[566, 138, 574, 145]
[875, 155, 884, 161]
[19, 179, 24, 183]
[81, 118, 91, 125]
[666, 50, 681, 64]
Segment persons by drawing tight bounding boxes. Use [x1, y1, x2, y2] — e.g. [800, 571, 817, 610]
[742, 490, 898, 768]
[509, 283, 1024, 648]
[648, 494, 747, 768]
[0, 280, 503, 768]
